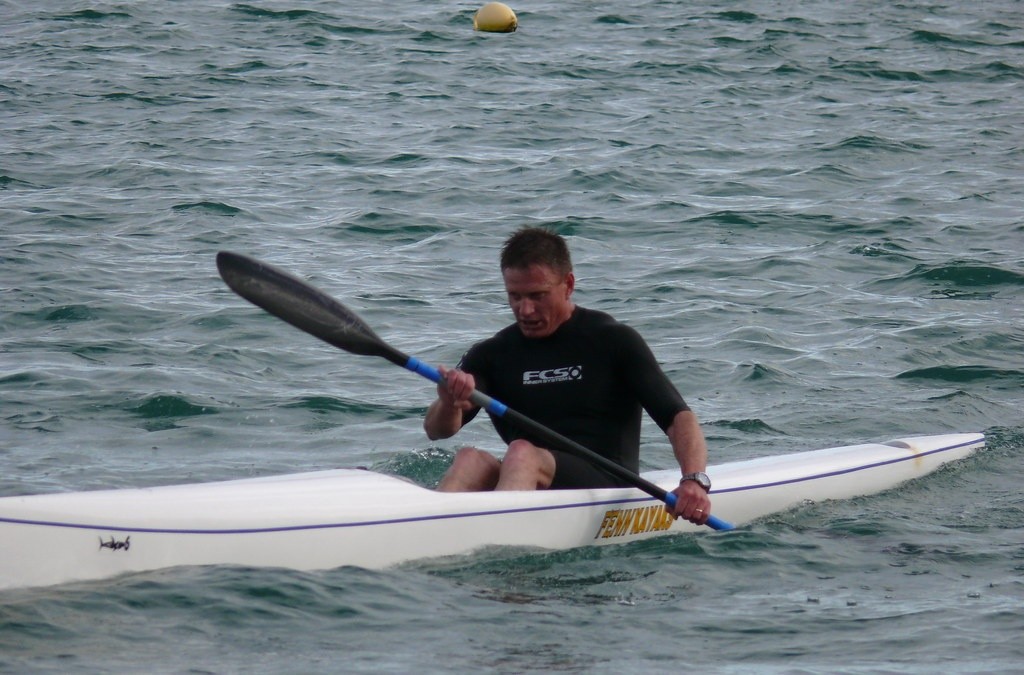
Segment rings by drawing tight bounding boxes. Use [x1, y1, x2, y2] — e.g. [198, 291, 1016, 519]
[696, 508, 703, 512]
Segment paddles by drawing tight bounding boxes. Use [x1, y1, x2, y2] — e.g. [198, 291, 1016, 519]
[215, 250, 737, 531]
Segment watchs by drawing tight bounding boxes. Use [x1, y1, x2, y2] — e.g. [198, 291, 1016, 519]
[679, 472, 711, 494]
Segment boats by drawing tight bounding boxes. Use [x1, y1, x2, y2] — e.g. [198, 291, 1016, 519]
[0, 429, 987, 586]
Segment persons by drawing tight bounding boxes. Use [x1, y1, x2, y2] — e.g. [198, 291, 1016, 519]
[423, 222, 712, 525]
